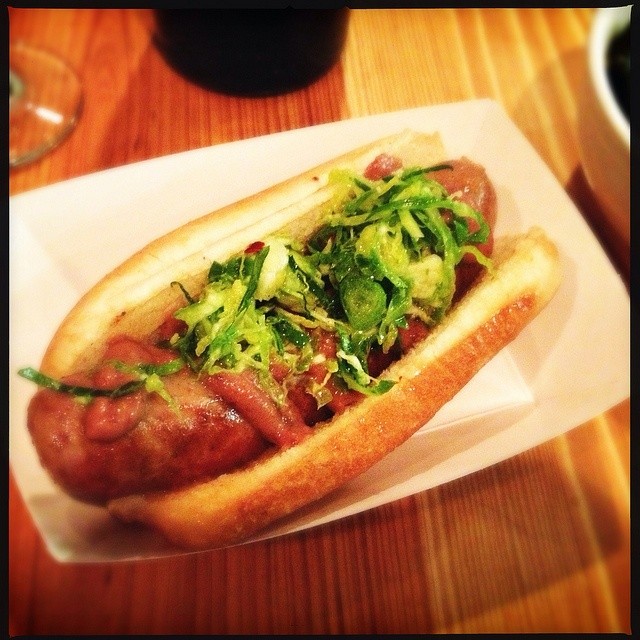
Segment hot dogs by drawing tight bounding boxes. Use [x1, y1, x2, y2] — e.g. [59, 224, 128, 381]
[19, 130, 565, 557]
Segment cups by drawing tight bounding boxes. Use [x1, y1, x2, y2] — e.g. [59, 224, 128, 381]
[580, 8, 631, 243]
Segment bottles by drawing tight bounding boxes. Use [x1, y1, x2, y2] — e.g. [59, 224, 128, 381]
[151, 5, 348, 97]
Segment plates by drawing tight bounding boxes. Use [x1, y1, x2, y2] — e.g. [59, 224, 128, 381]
[8, 99, 631, 565]
